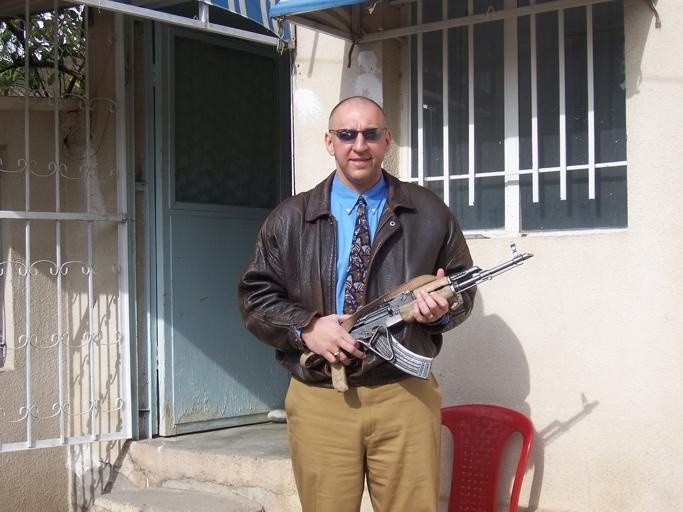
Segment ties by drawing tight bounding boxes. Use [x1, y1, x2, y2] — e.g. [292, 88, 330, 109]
[342, 193, 374, 315]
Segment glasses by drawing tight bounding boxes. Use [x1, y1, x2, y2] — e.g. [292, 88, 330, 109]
[328, 127, 390, 142]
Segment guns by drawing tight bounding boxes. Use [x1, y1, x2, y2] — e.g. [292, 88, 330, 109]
[306, 254, 533, 395]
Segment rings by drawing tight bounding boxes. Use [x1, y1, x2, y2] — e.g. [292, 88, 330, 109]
[334, 350, 342, 358]
[426, 314, 433, 319]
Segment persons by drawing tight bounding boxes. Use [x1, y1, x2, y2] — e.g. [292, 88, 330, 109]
[237, 96, 478, 512]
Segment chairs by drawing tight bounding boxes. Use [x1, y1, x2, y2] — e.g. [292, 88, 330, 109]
[440, 404, 535, 512]
